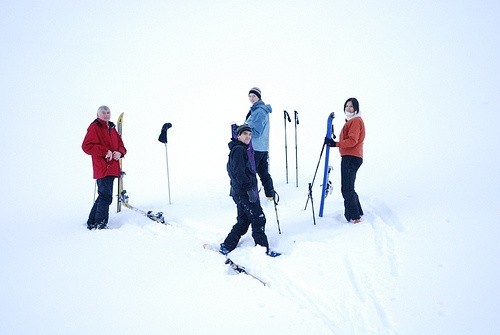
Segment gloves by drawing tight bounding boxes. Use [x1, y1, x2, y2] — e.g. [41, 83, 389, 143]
[247, 189, 258, 202]
[158, 123, 172, 143]
[324, 137, 336, 147]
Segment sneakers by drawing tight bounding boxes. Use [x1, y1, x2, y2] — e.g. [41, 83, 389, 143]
[219, 243, 230, 256]
[87, 223, 97, 230]
[95, 219, 110, 230]
[350, 218, 357, 223]
[356, 216, 361, 222]
[266, 248, 281, 257]
[267, 197, 274, 201]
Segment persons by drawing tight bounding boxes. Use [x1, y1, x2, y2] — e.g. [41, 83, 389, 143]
[325, 97, 366, 224]
[220, 123, 281, 258]
[241, 87, 276, 201]
[82, 106, 127, 230]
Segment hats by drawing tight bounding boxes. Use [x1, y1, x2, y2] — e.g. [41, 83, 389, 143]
[249, 87, 261, 98]
[237, 122, 252, 136]
